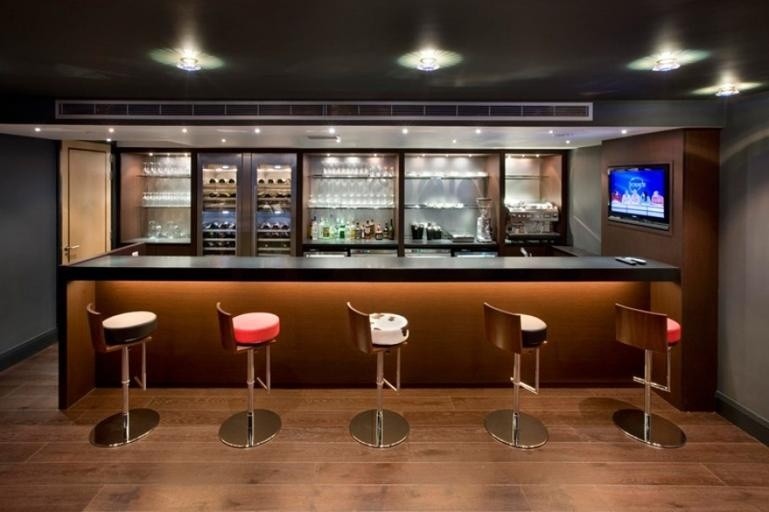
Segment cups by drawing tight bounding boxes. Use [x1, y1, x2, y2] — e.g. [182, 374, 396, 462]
[410, 223, 441, 240]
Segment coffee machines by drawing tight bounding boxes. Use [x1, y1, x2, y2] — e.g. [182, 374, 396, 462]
[505, 200, 564, 244]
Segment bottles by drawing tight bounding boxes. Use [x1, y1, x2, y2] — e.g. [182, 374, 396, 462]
[256, 178, 291, 213]
[309, 215, 393, 241]
[256, 220, 291, 255]
[208, 176, 236, 198]
[202, 220, 236, 256]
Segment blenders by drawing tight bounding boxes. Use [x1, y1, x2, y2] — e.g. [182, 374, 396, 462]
[475, 197, 493, 244]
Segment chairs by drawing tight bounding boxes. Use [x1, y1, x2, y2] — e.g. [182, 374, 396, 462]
[611, 298, 690, 450]
[212, 298, 282, 450]
[344, 295, 410, 450]
[480, 298, 550, 450]
[84, 298, 161, 450]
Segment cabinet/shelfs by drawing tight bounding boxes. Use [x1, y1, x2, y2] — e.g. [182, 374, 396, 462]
[401, 147, 503, 255]
[501, 147, 572, 258]
[299, 147, 402, 257]
[195, 145, 299, 258]
[114, 146, 194, 255]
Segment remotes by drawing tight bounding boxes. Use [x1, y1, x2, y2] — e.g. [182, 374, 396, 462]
[626, 257, 647, 264]
[616, 258, 637, 265]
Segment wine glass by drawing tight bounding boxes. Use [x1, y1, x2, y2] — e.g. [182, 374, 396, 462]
[141, 161, 189, 175]
[142, 191, 190, 206]
[306, 161, 395, 205]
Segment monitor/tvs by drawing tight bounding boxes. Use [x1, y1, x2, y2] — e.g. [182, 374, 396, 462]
[607, 163, 670, 231]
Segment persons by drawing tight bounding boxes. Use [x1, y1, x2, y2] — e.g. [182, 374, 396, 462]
[612, 188, 664, 205]
